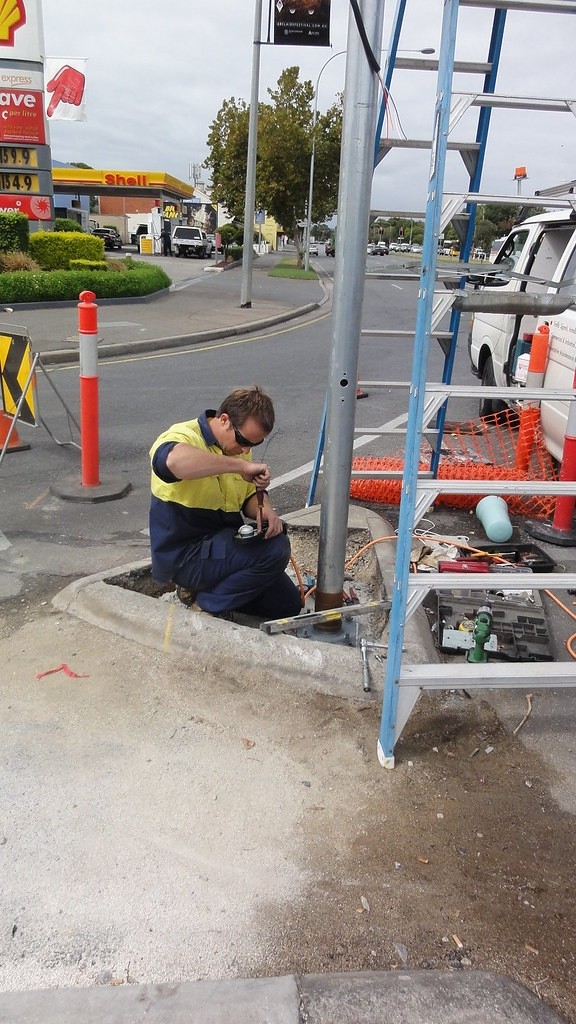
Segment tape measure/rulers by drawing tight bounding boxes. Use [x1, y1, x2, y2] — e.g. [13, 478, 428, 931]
[458, 619, 476, 632]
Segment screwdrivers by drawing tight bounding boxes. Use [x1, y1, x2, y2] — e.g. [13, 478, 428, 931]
[257, 491, 264, 533]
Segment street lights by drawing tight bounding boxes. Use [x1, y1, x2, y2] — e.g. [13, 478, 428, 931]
[305, 48, 435, 271]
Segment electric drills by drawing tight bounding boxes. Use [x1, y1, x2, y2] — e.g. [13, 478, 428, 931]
[467, 590, 493, 665]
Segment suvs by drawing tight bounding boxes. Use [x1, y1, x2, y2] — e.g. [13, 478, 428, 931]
[206, 234, 224, 254]
[172, 226, 211, 258]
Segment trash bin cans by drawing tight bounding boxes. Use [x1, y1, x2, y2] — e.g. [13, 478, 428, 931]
[253, 240, 273, 253]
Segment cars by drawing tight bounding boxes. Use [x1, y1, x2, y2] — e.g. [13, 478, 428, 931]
[309, 244, 318, 256]
[325, 241, 486, 259]
[91, 228, 122, 250]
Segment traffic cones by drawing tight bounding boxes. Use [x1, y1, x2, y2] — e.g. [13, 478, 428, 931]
[0, 378, 31, 453]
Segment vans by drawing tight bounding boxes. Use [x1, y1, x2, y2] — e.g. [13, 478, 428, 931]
[468, 208, 576, 464]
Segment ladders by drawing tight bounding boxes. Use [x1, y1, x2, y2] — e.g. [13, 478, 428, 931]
[307, 1, 576, 765]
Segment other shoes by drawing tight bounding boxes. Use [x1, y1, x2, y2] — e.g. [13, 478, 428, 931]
[177, 585, 194, 606]
[190, 601, 237, 624]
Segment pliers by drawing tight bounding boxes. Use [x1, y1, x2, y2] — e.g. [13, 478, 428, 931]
[343, 587, 360, 606]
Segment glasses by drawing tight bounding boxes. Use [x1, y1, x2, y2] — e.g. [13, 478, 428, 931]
[232, 424, 264, 447]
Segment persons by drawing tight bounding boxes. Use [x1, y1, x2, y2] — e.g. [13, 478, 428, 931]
[155, 228, 172, 258]
[204, 240, 215, 259]
[148, 385, 306, 622]
